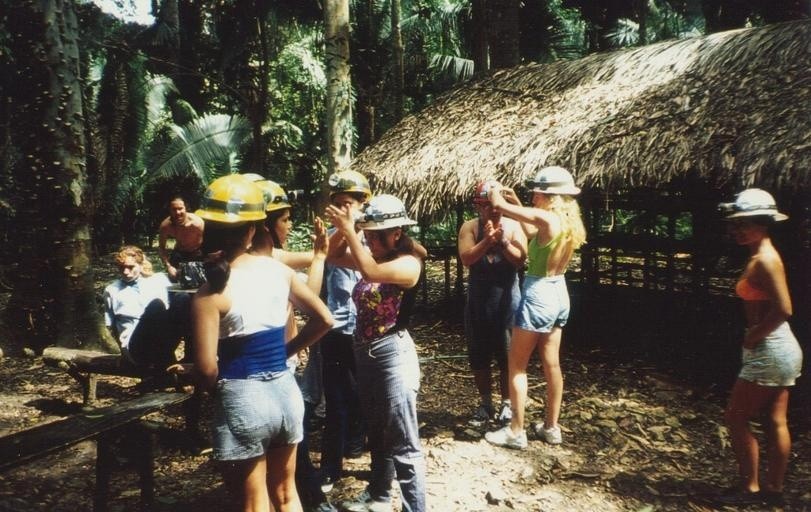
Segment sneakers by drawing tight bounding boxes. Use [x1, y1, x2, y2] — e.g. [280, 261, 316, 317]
[485, 399, 563, 450]
[721, 486, 765, 504]
[342, 492, 392, 512]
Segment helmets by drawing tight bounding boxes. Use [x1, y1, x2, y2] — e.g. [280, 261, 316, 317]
[528, 166, 581, 195]
[474, 180, 505, 202]
[719, 188, 790, 221]
[328, 170, 418, 230]
[194, 173, 292, 223]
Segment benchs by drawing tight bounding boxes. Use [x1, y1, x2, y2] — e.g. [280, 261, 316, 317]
[41, 347, 205, 406]
[0, 392, 194, 512]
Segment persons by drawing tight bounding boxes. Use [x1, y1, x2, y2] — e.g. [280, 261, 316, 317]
[458, 180, 528, 427]
[102, 170, 428, 512]
[717, 187, 804, 505]
[486, 166, 589, 451]
